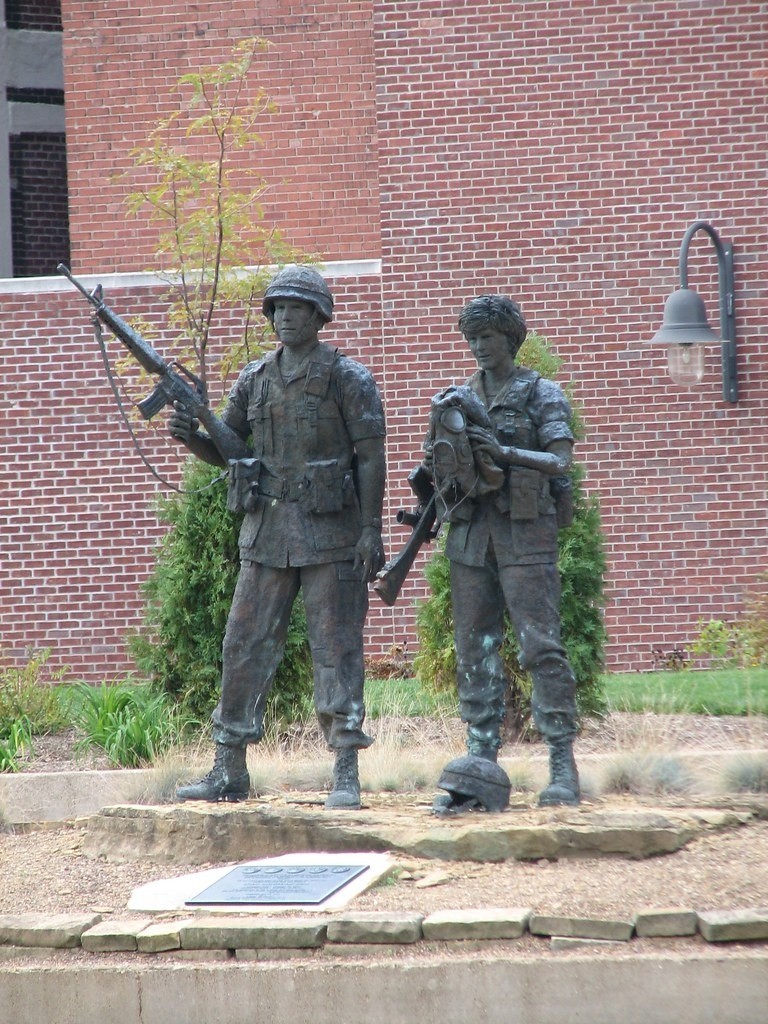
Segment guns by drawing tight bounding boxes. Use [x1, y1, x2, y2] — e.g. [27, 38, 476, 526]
[372, 465, 443, 607]
[57, 263, 250, 470]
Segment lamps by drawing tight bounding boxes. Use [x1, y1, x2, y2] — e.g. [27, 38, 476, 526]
[643, 221, 738, 402]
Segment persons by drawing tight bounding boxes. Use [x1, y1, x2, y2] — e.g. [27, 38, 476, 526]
[167, 266, 387, 810]
[423, 294, 580, 806]
